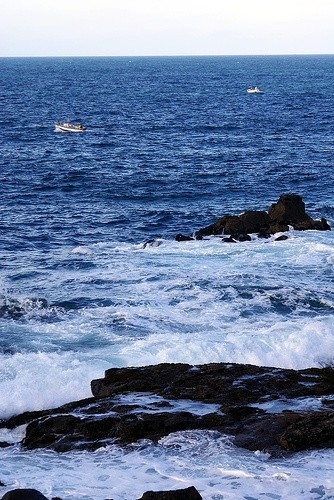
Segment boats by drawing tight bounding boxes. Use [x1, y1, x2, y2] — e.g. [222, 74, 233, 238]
[247, 86, 263, 94]
[55, 113, 85, 132]
